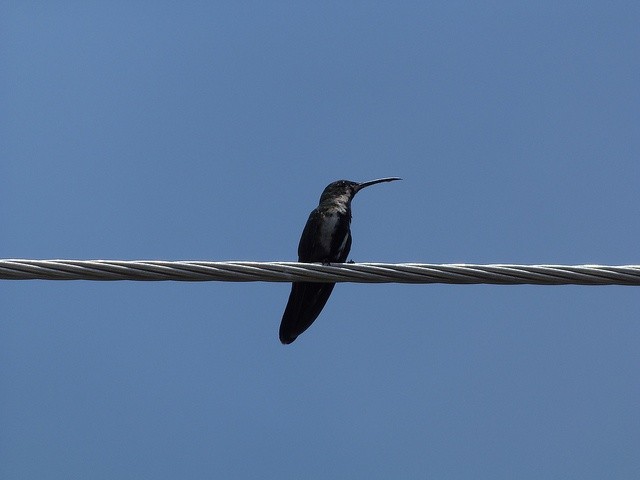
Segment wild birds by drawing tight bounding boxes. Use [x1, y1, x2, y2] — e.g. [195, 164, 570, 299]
[279, 176, 404, 344]
